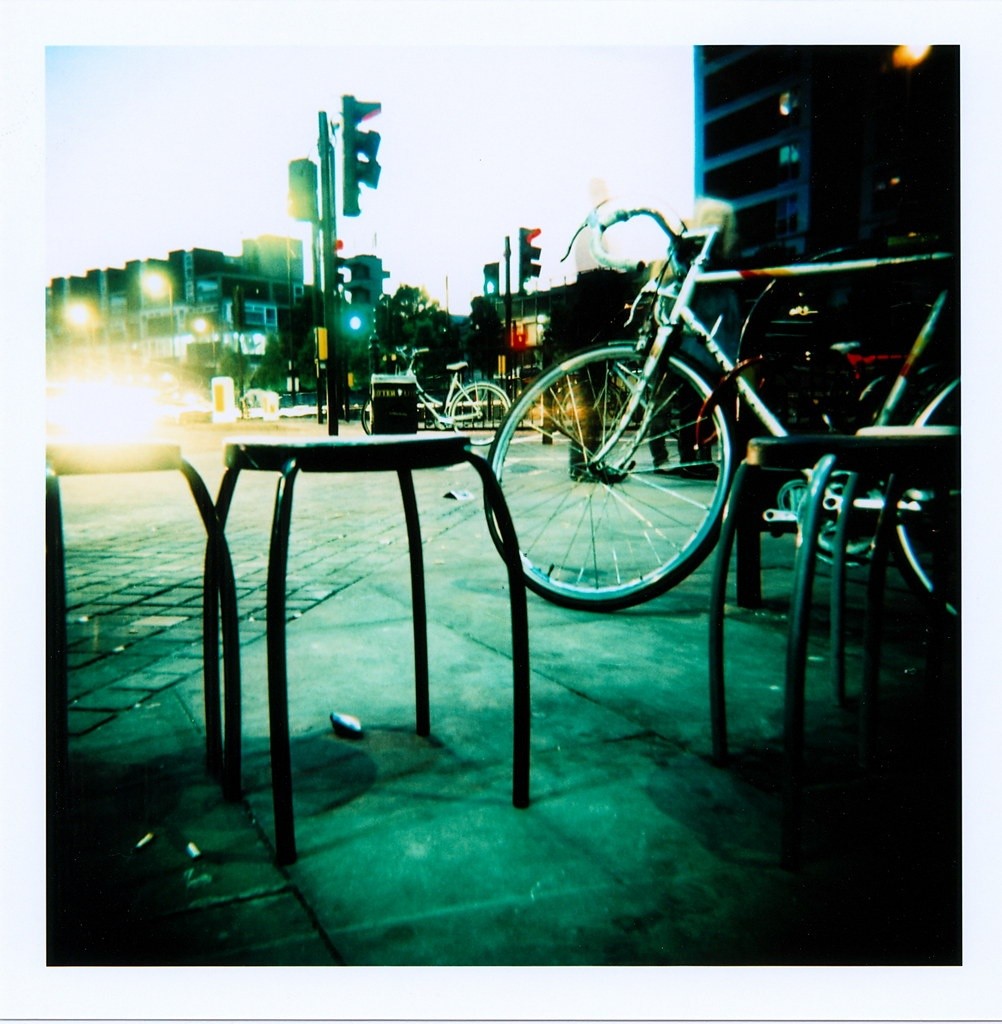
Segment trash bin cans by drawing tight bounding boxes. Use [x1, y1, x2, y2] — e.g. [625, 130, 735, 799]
[370, 373, 418, 435]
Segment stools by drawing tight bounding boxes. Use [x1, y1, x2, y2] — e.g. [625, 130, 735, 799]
[201, 434, 529, 868]
[46, 444, 243, 828]
[713, 426, 961, 870]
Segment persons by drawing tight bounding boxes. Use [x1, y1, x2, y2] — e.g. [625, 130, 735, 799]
[647, 368, 700, 467]
[545, 268, 634, 485]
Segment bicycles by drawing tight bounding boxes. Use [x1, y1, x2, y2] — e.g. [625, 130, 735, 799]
[481, 197, 962, 623]
[360, 344, 512, 447]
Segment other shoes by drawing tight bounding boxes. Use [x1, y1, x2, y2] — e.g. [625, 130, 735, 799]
[682, 459, 719, 480]
[657, 458, 679, 475]
[570, 463, 626, 486]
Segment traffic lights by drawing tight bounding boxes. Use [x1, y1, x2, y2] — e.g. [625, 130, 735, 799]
[482, 261, 500, 298]
[343, 93, 382, 217]
[517, 226, 542, 289]
